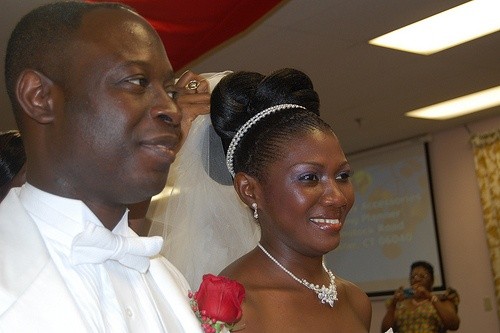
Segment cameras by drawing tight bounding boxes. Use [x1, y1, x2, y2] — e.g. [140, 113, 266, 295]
[403, 288, 417, 297]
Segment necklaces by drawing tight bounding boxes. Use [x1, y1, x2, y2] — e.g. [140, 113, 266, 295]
[256, 240, 339, 309]
[412, 298, 429, 306]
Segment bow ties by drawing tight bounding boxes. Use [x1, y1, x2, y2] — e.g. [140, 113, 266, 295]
[20, 189, 164, 274]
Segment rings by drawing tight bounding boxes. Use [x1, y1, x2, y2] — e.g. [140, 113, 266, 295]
[184, 80, 200, 94]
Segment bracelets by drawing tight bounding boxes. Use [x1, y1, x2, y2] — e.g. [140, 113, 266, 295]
[431, 295, 439, 303]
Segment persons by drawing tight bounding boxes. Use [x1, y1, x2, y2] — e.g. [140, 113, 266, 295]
[380, 260, 460, 333]
[0, 127, 27, 203]
[126, 70, 211, 237]
[0, 0, 204, 333]
[203, 67, 372, 333]
